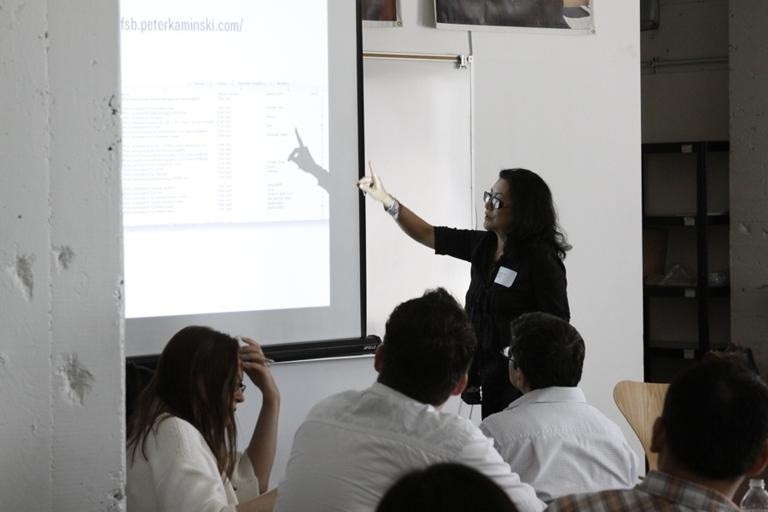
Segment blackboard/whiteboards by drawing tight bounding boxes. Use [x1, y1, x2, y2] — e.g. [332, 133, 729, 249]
[364, 51, 478, 366]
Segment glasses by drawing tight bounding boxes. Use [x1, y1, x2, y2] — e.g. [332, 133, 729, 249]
[482, 191, 512, 209]
[500, 346, 516, 363]
[233, 380, 246, 392]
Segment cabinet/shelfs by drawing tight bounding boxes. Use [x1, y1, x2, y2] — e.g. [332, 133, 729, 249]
[641, 137, 729, 384]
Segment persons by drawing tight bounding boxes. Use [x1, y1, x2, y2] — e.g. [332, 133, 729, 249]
[126, 326, 281, 512]
[542, 362, 768, 512]
[358, 158, 571, 420]
[274, 288, 548, 512]
[479, 312, 640, 502]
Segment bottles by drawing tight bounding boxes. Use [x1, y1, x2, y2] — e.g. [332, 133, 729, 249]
[739, 478, 768, 512]
